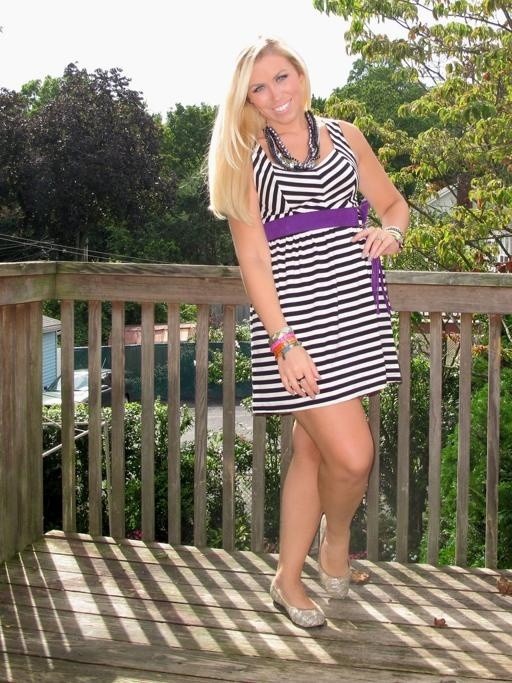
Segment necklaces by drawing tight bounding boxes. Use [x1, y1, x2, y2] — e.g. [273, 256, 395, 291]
[261, 108, 322, 171]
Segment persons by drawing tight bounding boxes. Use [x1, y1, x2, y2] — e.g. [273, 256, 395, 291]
[207, 33, 417, 632]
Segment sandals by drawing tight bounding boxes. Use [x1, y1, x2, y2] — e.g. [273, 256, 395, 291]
[270, 513, 352, 628]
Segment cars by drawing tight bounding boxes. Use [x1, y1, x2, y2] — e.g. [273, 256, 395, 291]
[42, 369, 129, 408]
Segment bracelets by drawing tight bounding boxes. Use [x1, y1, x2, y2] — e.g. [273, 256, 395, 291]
[382, 225, 406, 247]
[268, 324, 304, 360]
